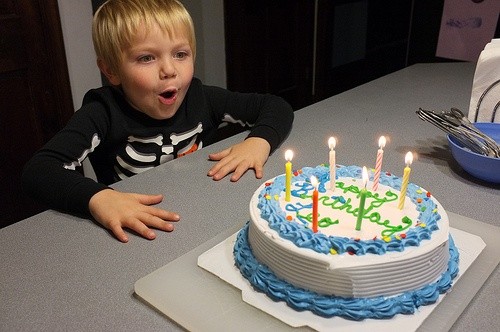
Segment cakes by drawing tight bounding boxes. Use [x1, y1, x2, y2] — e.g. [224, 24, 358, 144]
[232, 162, 459, 320]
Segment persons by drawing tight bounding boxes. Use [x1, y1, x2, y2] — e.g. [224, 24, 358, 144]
[20, 0, 294, 243]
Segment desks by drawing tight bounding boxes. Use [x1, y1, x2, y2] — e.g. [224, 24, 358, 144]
[0, 62, 500, 332]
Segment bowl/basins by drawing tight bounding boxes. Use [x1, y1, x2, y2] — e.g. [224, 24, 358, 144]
[447, 122, 500, 187]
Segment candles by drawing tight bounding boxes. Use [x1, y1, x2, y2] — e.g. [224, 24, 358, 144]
[371, 135, 387, 192]
[355, 165, 369, 231]
[328, 135, 337, 192]
[309, 174, 320, 233]
[284, 149, 294, 202]
[397, 150, 414, 211]
